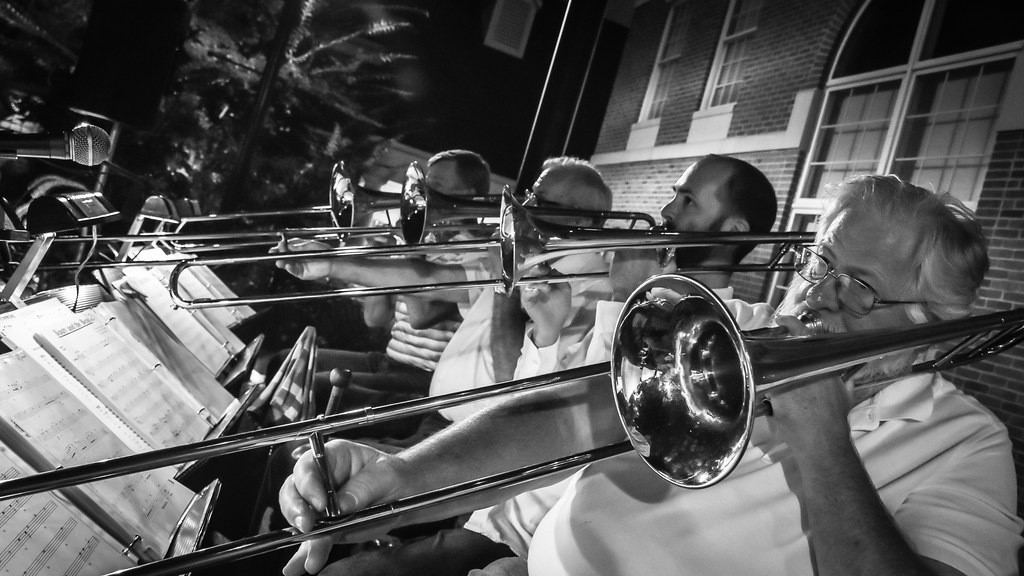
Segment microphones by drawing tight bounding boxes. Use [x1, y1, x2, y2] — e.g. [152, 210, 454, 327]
[1, 124, 110, 167]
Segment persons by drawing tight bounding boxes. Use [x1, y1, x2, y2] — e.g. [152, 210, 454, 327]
[278, 171, 1022, 576]
[268, 148, 778, 576]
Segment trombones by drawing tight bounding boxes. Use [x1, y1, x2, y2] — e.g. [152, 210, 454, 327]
[1, 272, 1022, 576]
[167, 182, 818, 311]
[173, 157, 531, 247]
[170, 160, 658, 254]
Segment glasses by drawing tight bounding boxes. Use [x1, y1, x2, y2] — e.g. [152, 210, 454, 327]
[793, 244, 937, 317]
[523, 189, 569, 208]
